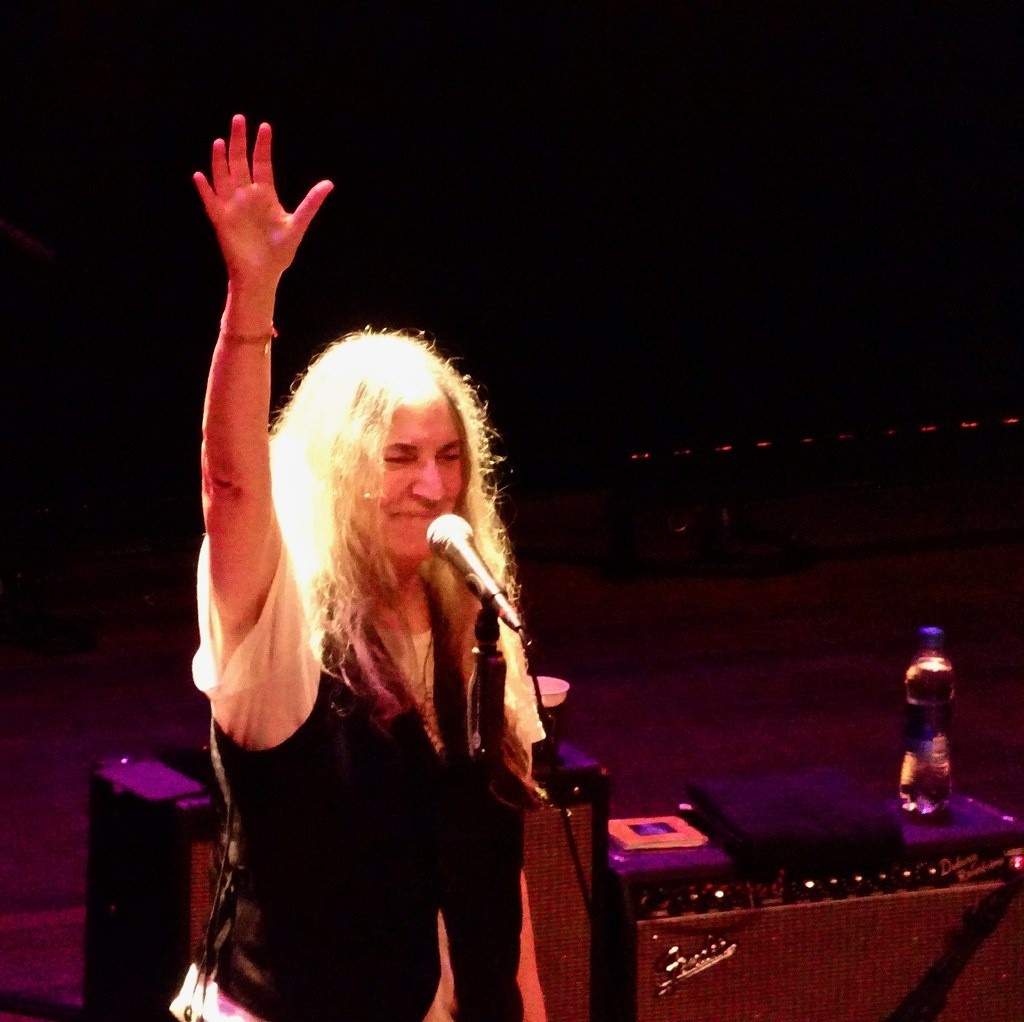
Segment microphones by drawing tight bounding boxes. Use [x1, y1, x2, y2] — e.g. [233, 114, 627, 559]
[424, 515, 523, 634]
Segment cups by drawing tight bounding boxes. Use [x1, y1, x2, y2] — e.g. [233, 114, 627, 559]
[528, 673, 570, 774]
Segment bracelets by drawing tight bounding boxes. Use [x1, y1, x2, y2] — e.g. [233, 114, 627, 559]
[218, 311, 276, 342]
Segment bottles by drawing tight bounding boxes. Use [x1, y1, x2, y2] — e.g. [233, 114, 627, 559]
[897, 628, 956, 817]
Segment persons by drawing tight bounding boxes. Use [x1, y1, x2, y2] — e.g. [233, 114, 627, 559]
[166, 114, 550, 1021]
